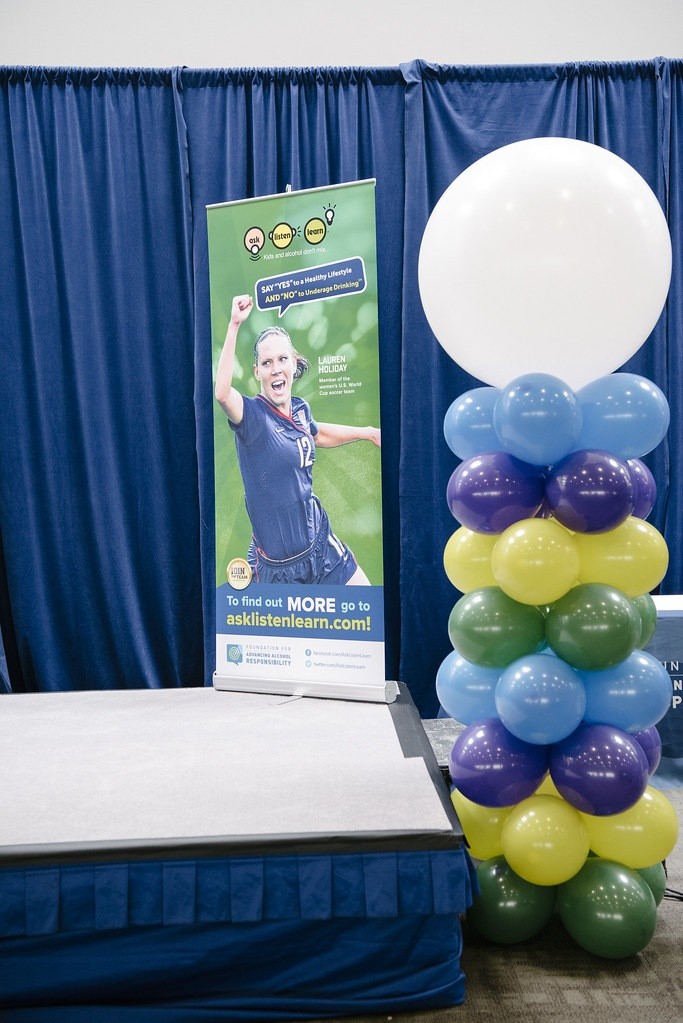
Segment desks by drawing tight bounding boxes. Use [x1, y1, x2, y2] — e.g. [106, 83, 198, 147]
[0, 680, 480, 1023]
[641, 594, 683, 759]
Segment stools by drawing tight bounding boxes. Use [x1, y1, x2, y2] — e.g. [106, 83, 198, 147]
[420, 717, 467, 792]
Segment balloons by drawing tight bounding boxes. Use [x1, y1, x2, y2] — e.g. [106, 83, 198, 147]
[417, 135, 674, 962]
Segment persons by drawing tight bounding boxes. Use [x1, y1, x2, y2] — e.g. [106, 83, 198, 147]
[214, 294, 380, 586]
